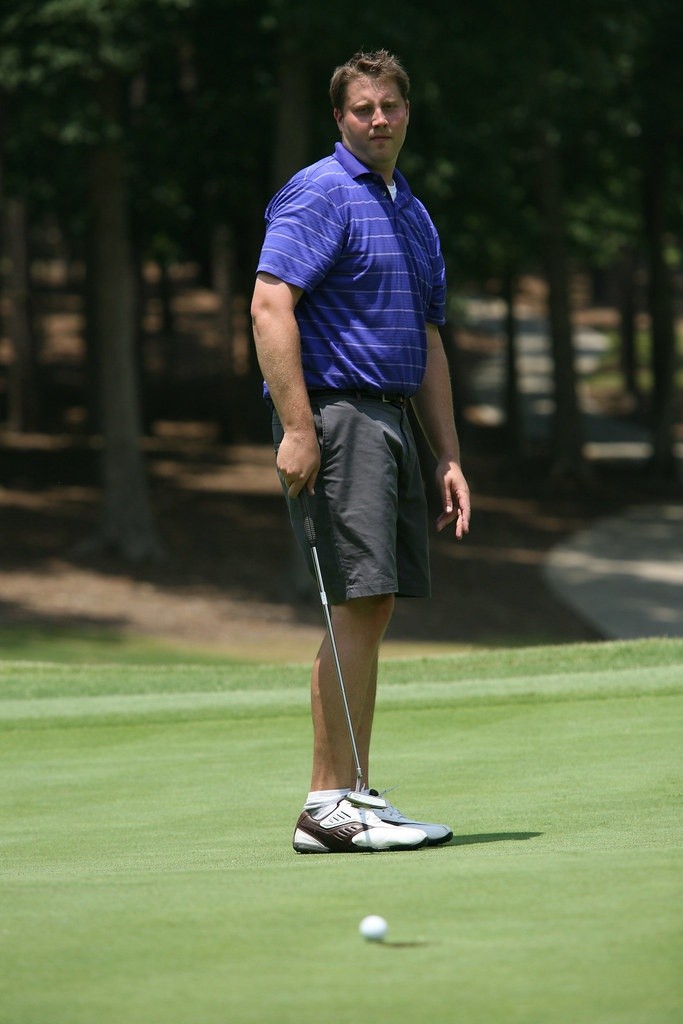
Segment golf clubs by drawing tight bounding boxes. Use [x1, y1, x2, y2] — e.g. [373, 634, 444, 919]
[273, 442, 389, 810]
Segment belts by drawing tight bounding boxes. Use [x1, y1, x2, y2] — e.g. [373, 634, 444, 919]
[304, 388, 408, 403]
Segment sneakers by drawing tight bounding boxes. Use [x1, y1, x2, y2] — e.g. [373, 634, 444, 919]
[292, 795, 429, 854]
[368, 788, 454, 846]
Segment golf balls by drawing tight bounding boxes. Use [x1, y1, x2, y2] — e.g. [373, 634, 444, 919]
[358, 914, 389, 941]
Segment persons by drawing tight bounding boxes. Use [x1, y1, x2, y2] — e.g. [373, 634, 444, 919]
[251, 47, 473, 855]
